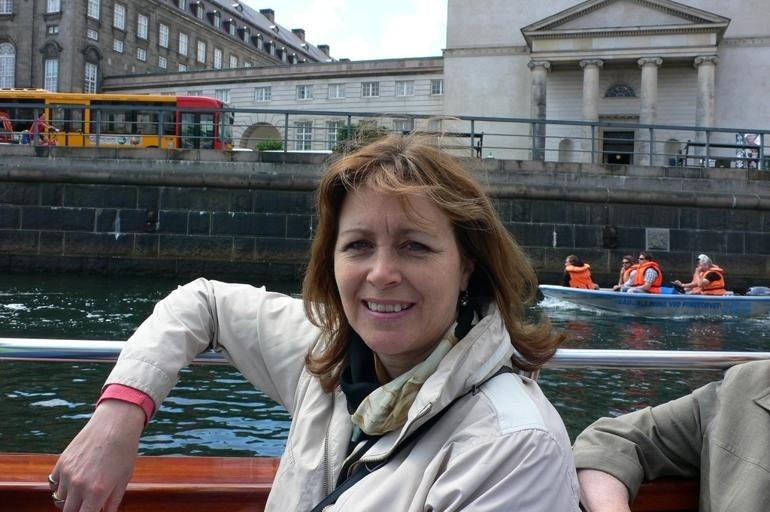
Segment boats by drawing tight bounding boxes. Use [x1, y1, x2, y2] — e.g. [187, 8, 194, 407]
[538, 283, 770, 321]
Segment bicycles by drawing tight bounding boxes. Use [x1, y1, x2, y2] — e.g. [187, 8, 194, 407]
[18, 127, 60, 145]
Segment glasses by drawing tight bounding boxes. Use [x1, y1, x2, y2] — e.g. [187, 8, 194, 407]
[622, 257, 643, 265]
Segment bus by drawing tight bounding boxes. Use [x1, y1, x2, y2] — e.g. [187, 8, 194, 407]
[0, 87, 236, 151]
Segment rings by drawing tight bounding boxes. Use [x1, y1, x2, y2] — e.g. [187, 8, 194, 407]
[51, 492, 64, 503]
[48, 474, 58, 486]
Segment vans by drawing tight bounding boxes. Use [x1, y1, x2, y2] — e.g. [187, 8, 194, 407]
[0, 110, 15, 144]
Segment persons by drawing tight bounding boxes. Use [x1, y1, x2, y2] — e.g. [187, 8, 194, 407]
[562, 255, 596, 289]
[49, 132, 581, 512]
[571, 358, 769, 512]
[613, 252, 662, 293]
[671, 254, 726, 296]
[29, 112, 54, 146]
